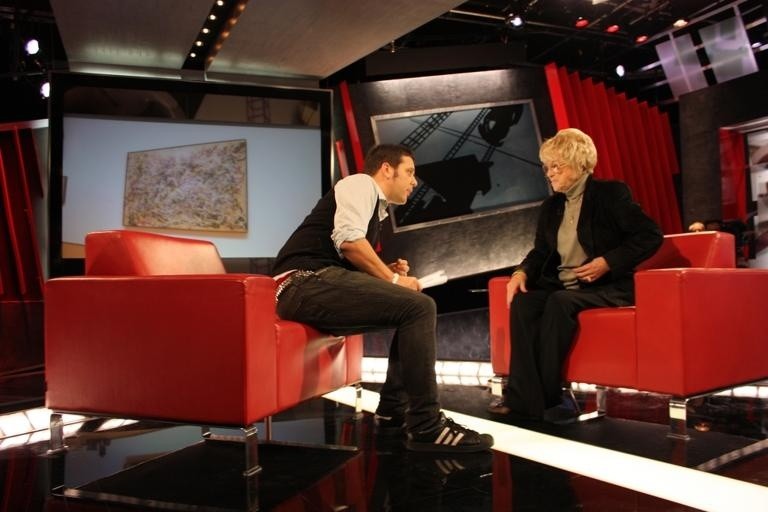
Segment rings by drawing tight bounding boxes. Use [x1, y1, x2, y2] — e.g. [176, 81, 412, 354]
[587, 275, 592, 282]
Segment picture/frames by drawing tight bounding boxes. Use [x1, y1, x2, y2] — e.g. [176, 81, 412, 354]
[121, 139, 250, 237]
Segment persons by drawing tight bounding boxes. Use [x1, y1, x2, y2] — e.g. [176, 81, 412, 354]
[272, 143, 495, 454]
[485, 128, 664, 423]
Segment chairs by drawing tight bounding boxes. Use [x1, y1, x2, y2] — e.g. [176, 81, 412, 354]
[42, 229, 363, 478]
[488, 231, 768, 443]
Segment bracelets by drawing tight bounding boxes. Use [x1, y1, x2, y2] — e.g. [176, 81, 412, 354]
[391, 272, 400, 284]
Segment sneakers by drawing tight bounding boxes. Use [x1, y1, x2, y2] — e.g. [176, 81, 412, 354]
[372, 411, 494, 454]
